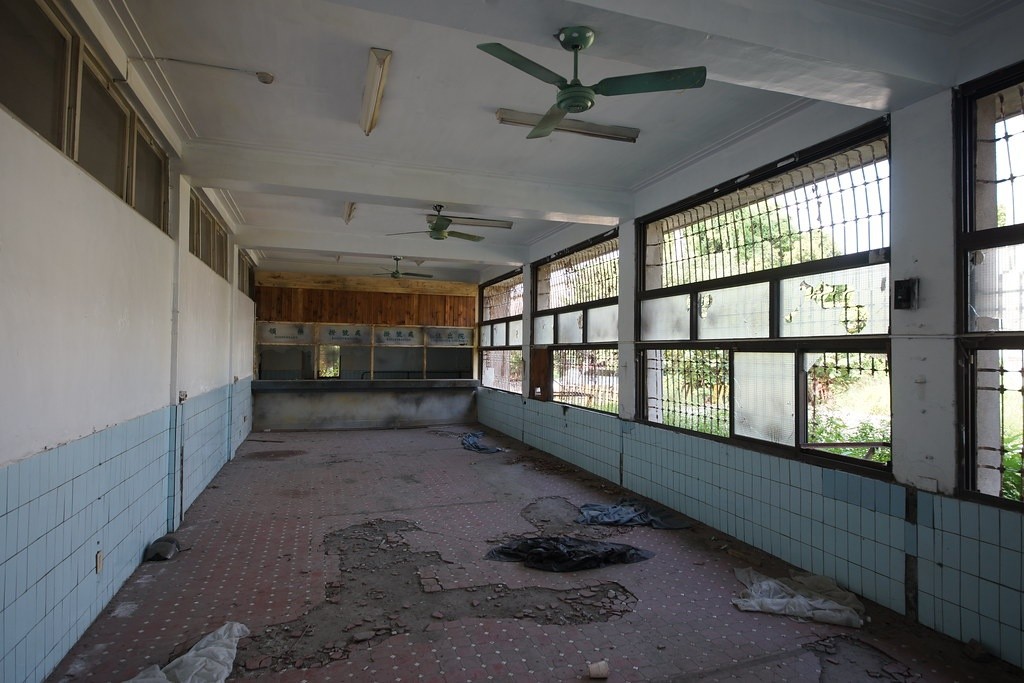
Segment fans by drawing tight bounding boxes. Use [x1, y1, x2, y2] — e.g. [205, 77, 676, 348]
[477, 25, 707, 139]
[371, 256, 434, 279]
[385, 205, 486, 242]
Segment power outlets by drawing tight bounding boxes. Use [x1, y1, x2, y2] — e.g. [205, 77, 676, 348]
[96, 550, 103, 573]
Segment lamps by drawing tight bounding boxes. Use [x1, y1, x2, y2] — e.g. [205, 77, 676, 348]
[494, 107, 640, 143]
[341, 201, 359, 225]
[358, 47, 393, 136]
[426, 215, 514, 230]
